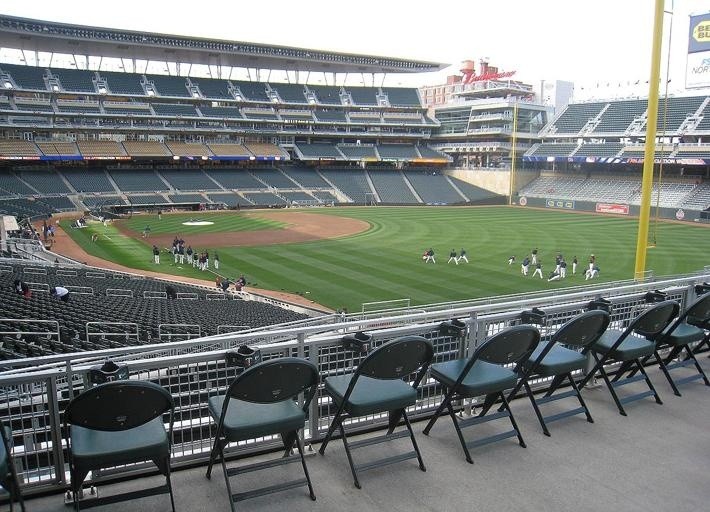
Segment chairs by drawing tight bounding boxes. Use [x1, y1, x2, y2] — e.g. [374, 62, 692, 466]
[206, 357, 320, 512]
[423, 324, 541, 464]
[320, 336, 434, 488]
[1, 420, 26, 512]
[64, 380, 174, 511]
[578, 300, 680, 415]
[627, 292, 709, 386]
[498, 309, 610, 437]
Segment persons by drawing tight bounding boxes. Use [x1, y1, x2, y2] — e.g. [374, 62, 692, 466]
[51, 286, 68, 302]
[198, 201, 229, 211]
[215, 274, 247, 294]
[143, 224, 150, 237]
[341, 307, 348, 322]
[423, 248, 436, 264]
[21, 222, 57, 240]
[448, 248, 469, 265]
[508, 255, 514, 265]
[548, 253, 601, 281]
[521, 246, 544, 280]
[97, 213, 114, 227]
[158, 210, 162, 220]
[71, 217, 85, 229]
[91, 233, 97, 242]
[14, 280, 31, 297]
[153, 245, 160, 264]
[172, 236, 219, 271]
